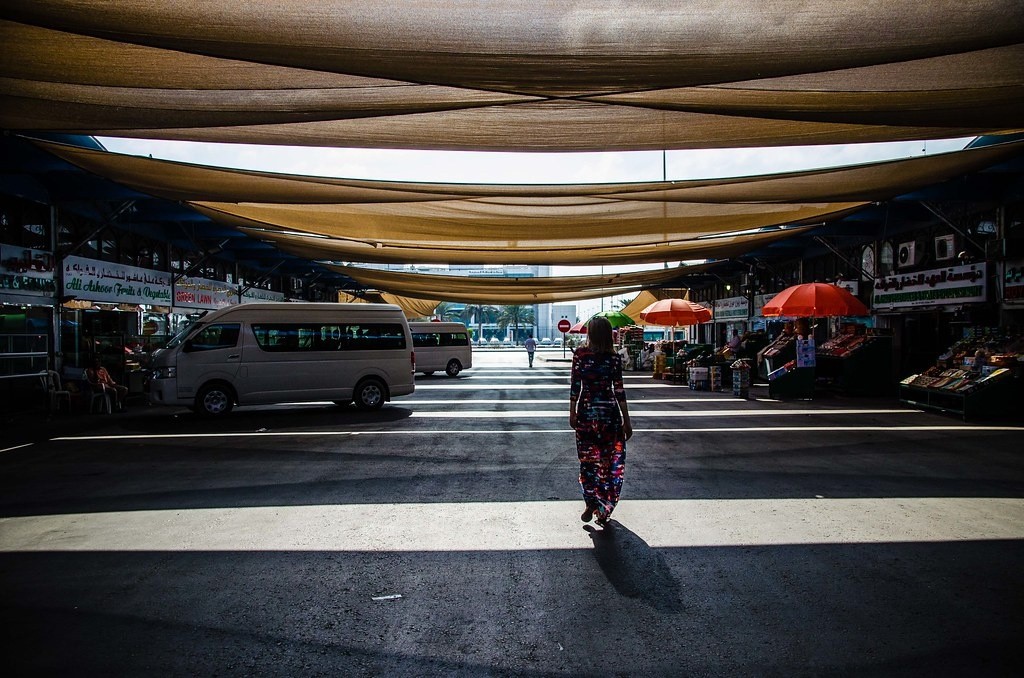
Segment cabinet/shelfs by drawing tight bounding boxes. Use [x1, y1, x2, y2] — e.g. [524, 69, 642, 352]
[94, 336, 175, 393]
[815, 323, 892, 399]
[757, 332, 799, 380]
[899, 338, 1024, 424]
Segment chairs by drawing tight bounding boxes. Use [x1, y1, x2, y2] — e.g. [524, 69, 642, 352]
[84, 368, 122, 415]
[39, 370, 71, 416]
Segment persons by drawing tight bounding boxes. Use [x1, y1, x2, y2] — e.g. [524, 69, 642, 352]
[85, 353, 127, 415]
[569, 316, 633, 528]
[727, 328, 741, 360]
[525, 334, 536, 367]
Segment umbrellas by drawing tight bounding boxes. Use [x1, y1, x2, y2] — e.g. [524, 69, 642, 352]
[569, 320, 589, 334]
[640, 298, 713, 376]
[583, 310, 635, 329]
[760, 283, 870, 337]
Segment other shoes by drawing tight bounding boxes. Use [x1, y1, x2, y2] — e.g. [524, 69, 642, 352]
[599, 518, 606, 523]
[582, 503, 598, 522]
[529, 364, 532, 367]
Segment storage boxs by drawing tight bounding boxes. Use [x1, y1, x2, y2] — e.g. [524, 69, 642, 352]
[690, 367, 707, 380]
[733, 369, 749, 398]
[688, 380, 709, 390]
[708, 366, 722, 392]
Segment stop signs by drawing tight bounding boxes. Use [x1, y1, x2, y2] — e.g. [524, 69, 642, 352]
[558, 320, 571, 332]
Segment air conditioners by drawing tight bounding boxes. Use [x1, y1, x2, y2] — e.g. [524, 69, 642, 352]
[898, 241, 919, 268]
[935, 234, 956, 261]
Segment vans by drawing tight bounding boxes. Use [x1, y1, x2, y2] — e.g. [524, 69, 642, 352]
[408, 321, 472, 377]
[144, 302, 416, 419]
